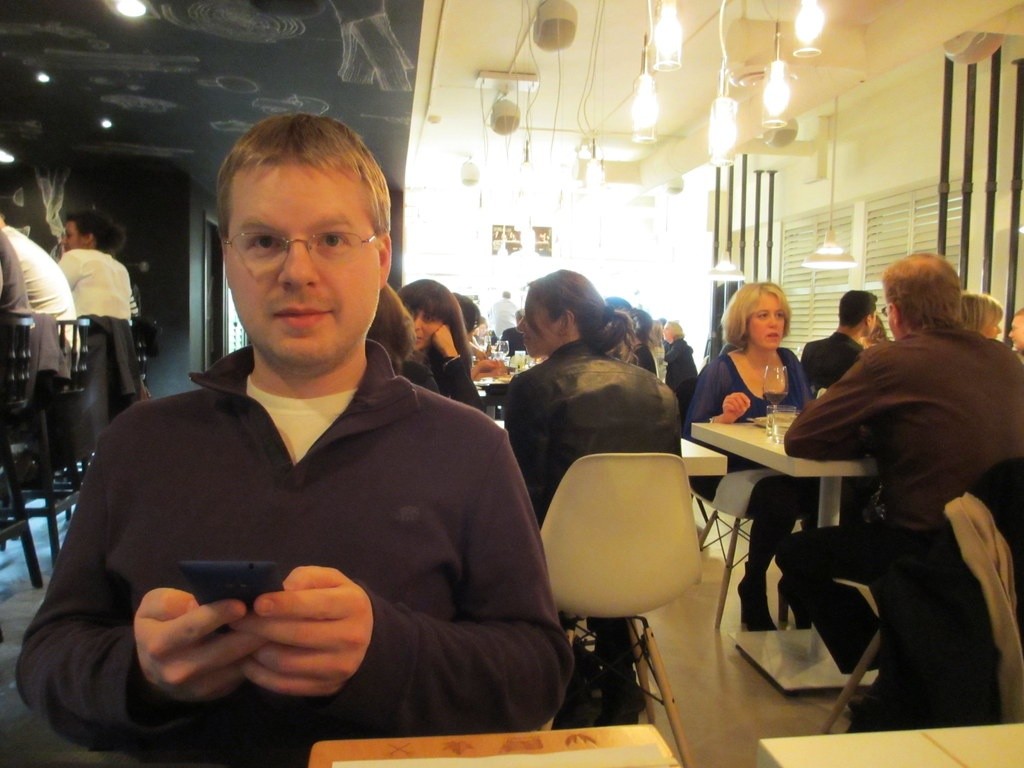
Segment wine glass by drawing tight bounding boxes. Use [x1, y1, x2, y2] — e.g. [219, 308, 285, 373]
[763, 365, 789, 440]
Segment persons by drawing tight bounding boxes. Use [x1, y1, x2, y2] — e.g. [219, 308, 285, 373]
[16, 106, 574, 768]
[364, 279, 441, 395]
[0, 211, 142, 416]
[397, 279, 499, 415]
[681, 278, 847, 631]
[489, 289, 518, 341]
[785, 251, 1024, 678]
[801, 289, 887, 399]
[1008, 309, 1024, 357]
[959, 289, 1005, 340]
[504, 268, 698, 731]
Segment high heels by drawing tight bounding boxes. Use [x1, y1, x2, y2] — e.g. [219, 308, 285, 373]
[777, 574, 811, 629]
[739, 561, 777, 632]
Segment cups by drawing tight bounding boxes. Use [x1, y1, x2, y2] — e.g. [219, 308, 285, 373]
[766, 405, 796, 445]
[470, 332, 546, 377]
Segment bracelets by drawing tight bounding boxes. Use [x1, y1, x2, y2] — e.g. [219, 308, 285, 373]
[710, 416, 716, 424]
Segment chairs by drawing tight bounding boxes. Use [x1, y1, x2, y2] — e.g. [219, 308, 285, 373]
[537, 451, 704, 768]
[0, 315, 149, 646]
[690, 488, 753, 631]
[822, 579, 879, 733]
[945, 491, 1024, 724]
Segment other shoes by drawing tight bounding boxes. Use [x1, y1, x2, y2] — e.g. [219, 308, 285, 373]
[593, 635, 646, 727]
[550, 636, 609, 731]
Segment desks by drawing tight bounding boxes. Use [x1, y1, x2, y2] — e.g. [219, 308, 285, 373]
[690, 416, 879, 700]
[754, 722, 1024, 768]
[307, 725, 682, 768]
[476, 376, 512, 420]
[680, 437, 728, 479]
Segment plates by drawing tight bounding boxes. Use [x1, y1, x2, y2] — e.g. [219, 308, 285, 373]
[746, 414, 801, 428]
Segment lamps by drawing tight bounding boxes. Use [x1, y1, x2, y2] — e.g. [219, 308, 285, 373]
[585, 0, 605, 194]
[707, 249, 746, 283]
[792, 0, 826, 58]
[708, 0, 739, 168]
[631, 0, 661, 144]
[802, 96, 857, 271]
[652, 0, 682, 73]
[760, 22, 792, 130]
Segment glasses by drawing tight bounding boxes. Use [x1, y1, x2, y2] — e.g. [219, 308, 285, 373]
[882, 303, 897, 317]
[223, 230, 381, 272]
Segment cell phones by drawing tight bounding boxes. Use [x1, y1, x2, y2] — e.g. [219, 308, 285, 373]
[178, 560, 284, 635]
